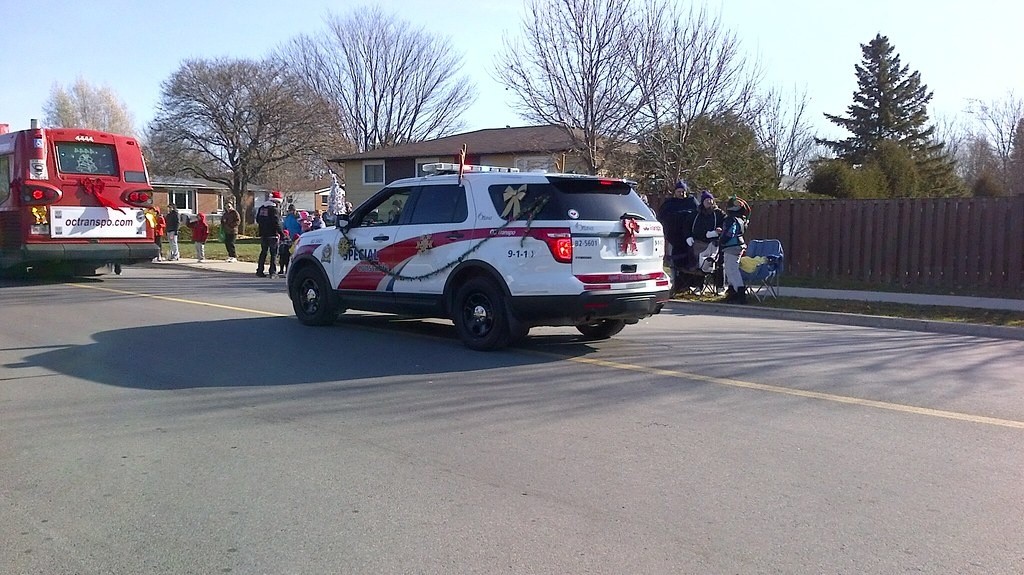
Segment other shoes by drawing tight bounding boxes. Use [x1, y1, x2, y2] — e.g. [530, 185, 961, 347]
[722, 294, 747, 304]
[694, 290, 705, 296]
[169, 257, 179, 261]
[256, 271, 266, 277]
[225, 257, 237, 262]
[278, 271, 284, 274]
[198, 259, 205, 262]
[270, 273, 280, 279]
[717, 288, 726, 296]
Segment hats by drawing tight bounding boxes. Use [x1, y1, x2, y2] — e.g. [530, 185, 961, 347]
[288, 204, 296, 211]
[298, 211, 309, 220]
[283, 230, 289, 237]
[701, 191, 713, 204]
[675, 181, 686, 191]
[167, 203, 175, 208]
[392, 200, 402, 208]
[293, 233, 300, 241]
[269, 191, 283, 203]
[155, 206, 160, 214]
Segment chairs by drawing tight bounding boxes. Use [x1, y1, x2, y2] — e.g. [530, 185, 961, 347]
[673, 256, 718, 301]
[738, 239, 784, 304]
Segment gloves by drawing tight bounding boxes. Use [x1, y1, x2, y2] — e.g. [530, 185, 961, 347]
[686, 237, 694, 246]
[706, 230, 718, 238]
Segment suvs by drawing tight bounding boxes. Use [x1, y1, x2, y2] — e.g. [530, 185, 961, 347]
[285, 163, 672, 352]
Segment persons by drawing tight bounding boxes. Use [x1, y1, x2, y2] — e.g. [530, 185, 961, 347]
[142, 203, 167, 262]
[656, 179, 699, 299]
[714, 199, 750, 304]
[369, 200, 404, 226]
[221, 202, 241, 264]
[186, 213, 210, 262]
[682, 190, 728, 296]
[278, 202, 353, 278]
[256, 191, 290, 279]
[166, 202, 181, 261]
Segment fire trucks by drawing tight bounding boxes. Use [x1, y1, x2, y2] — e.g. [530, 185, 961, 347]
[0, 129, 160, 281]
